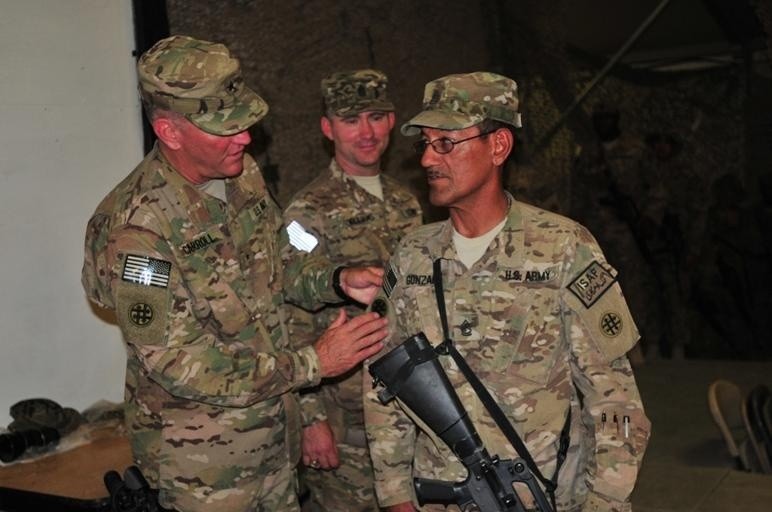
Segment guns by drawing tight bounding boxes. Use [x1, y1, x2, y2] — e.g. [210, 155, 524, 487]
[0, 465, 170, 512]
[368, 332, 552, 512]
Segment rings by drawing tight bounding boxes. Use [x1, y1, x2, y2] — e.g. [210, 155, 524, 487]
[309, 456, 321, 470]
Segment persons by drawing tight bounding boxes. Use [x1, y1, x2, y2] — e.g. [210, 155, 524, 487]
[364, 72, 649, 512]
[281, 68, 423, 512]
[81, 35, 389, 512]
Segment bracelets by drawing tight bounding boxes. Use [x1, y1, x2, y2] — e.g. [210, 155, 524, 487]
[332, 267, 349, 300]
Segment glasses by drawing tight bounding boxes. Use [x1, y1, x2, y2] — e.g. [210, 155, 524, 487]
[414, 130, 496, 154]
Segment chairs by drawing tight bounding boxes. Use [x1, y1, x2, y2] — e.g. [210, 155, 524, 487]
[707, 376, 771, 475]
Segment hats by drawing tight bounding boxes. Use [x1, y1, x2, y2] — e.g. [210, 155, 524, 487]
[400, 72, 522, 136]
[321, 69, 396, 117]
[137, 36, 269, 136]
